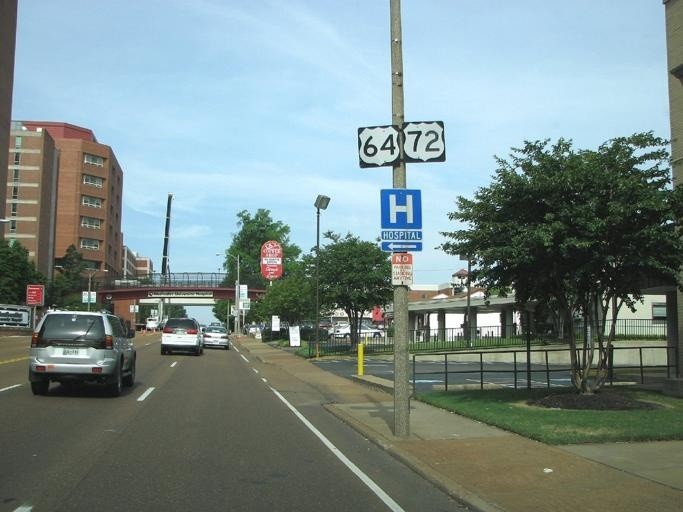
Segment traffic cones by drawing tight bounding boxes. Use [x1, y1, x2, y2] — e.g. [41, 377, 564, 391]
[140, 327, 161, 337]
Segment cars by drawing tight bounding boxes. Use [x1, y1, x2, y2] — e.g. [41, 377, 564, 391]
[241, 319, 390, 341]
[144, 317, 231, 355]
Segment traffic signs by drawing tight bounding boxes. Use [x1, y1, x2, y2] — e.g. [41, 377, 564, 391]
[380, 231, 423, 252]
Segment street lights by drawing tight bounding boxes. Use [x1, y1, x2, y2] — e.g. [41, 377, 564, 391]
[312, 193, 331, 361]
[87, 268, 111, 312]
[214, 251, 242, 338]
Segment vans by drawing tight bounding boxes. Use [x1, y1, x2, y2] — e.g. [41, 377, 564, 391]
[25, 307, 141, 398]
